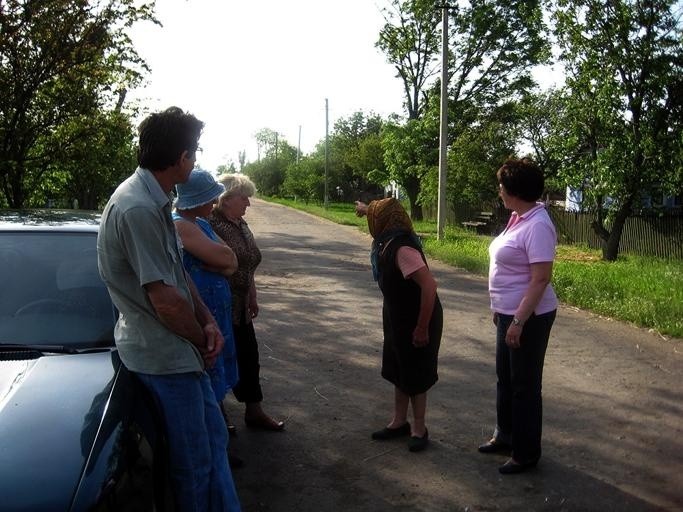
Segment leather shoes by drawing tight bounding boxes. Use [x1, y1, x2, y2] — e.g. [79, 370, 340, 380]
[245, 413, 284, 432]
[227, 424, 235, 433]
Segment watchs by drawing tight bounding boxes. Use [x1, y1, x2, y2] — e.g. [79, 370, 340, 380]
[513, 318, 524, 326]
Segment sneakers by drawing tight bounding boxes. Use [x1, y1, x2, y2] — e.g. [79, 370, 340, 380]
[498, 456, 537, 474]
[372, 422, 410, 439]
[407, 426, 428, 451]
[478, 437, 513, 453]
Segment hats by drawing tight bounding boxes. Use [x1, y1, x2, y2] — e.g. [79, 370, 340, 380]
[173, 170, 225, 211]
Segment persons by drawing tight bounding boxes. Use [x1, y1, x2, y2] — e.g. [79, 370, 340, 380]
[171, 170, 245, 470]
[478, 160, 559, 474]
[354, 198, 443, 452]
[97, 106, 241, 512]
[207, 174, 285, 433]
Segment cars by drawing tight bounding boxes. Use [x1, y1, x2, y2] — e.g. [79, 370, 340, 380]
[0, 208, 181, 512]
